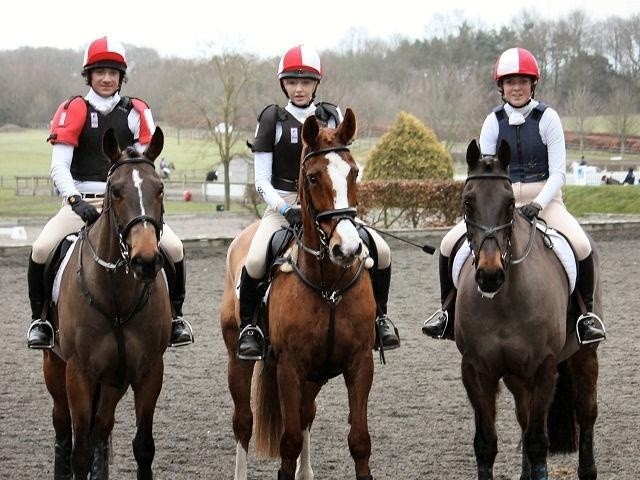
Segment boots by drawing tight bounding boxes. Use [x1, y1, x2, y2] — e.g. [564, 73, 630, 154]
[370, 261, 400, 347]
[238, 266, 268, 356]
[422, 250, 457, 340]
[28, 250, 53, 350]
[160, 247, 195, 344]
[575, 250, 605, 347]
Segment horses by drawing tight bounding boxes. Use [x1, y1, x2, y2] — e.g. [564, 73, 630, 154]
[217, 105, 384, 480]
[42, 124, 174, 479]
[157, 162, 176, 181]
[602, 175, 623, 185]
[450, 137, 601, 480]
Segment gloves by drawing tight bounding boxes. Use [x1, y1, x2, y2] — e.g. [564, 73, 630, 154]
[68, 195, 99, 226]
[520, 202, 542, 221]
[284, 207, 302, 229]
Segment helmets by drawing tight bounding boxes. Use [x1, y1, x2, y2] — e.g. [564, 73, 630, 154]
[494, 47, 540, 84]
[83, 36, 127, 76]
[278, 45, 322, 83]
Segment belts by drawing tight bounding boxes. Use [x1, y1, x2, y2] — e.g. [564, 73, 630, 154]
[81, 193, 104, 199]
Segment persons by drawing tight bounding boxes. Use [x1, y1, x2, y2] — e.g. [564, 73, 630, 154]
[234, 45, 401, 355]
[159, 157, 170, 177]
[418, 46, 605, 340]
[623, 167, 635, 184]
[601, 175, 619, 183]
[580, 156, 586, 165]
[28, 36, 190, 347]
